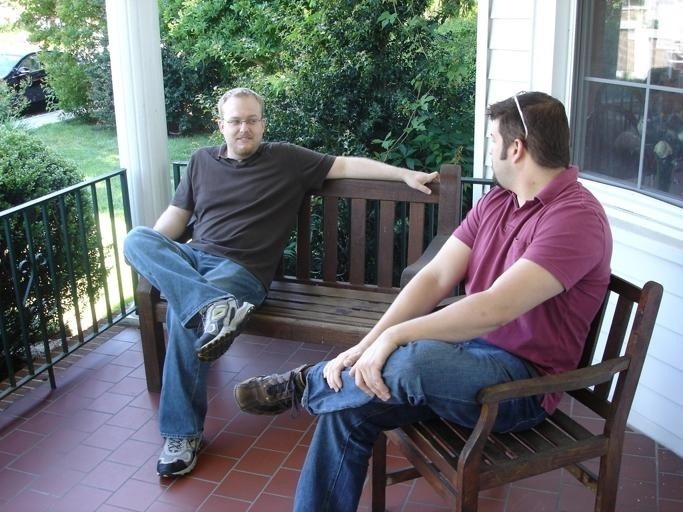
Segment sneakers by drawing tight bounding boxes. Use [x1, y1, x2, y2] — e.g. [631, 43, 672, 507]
[193, 296, 255, 361]
[233, 363, 315, 416]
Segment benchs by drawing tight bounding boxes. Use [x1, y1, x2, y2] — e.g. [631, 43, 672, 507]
[135, 163, 462, 393]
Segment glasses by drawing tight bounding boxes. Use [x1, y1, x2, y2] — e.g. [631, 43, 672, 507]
[512, 90, 528, 142]
[224, 117, 263, 126]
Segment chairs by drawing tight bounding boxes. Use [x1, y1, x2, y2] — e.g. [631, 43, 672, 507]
[372, 276, 663, 511]
[583, 83, 644, 180]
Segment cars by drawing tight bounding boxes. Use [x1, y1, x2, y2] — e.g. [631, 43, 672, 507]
[0, 46, 85, 123]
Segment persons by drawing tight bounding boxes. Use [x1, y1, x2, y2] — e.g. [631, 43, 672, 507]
[122, 89, 438, 478]
[233, 92, 612, 512]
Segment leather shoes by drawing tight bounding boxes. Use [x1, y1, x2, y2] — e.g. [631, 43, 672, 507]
[157, 430, 208, 477]
